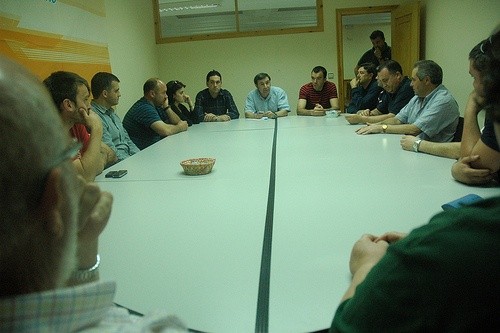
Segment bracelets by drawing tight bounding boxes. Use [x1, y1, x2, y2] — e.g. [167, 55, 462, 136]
[190, 105, 193, 107]
[70, 255, 100, 282]
[163, 105, 171, 111]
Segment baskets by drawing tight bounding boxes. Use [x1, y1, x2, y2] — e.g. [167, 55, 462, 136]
[180, 157, 216, 175]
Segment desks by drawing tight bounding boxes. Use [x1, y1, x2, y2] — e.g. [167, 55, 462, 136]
[93, 116, 500, 333]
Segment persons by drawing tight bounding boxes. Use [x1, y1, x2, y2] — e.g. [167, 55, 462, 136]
[166, 81, 202, 127]
[42, 72, 116, 182]
[0, 60, 203, 333]
[354, 60, 459, 142]
[452, 32, 500, 184]
[193, 70, 240, 122]
[328, 193, 500, 333]
[122, 71, 188, 150]
[357, 59, 415, 125]
[343, 30, 391, 114]
[296, 65, 339, 116]
[243, 73, 290, 118]
[401, 135, 461, 158]
[90, 72, 140, 162]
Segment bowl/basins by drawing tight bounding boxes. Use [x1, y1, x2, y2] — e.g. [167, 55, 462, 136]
[325, 110, 341, 118]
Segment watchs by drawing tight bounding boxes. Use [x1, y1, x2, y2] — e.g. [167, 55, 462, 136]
[381, 124, 388, 134]
[413, 139, 421, 152]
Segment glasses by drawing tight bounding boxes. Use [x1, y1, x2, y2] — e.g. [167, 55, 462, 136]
[407, 76, 424, 81]
[172, 80, 179, 88]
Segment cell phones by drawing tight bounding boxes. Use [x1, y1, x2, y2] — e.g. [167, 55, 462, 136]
[106, 170, 127, 178]
